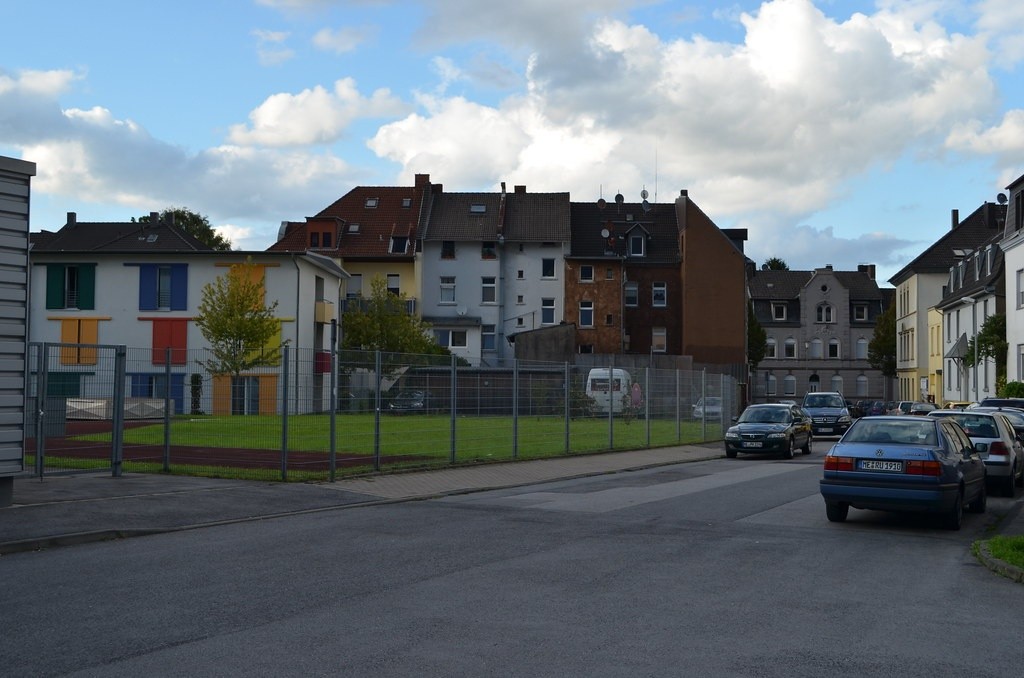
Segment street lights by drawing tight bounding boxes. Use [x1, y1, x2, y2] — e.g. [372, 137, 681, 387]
[961, 296, 979, 401]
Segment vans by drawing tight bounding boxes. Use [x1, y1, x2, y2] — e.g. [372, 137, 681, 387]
[585, 368, 633, 416]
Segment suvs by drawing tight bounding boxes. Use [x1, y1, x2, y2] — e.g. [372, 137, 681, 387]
[801, 390, 854, 436]
[980, 397, 1024, 409]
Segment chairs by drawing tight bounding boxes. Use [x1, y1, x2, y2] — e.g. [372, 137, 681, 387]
[975, 424, 996, 437]
[924, 430, 936, 445]
[869, 431, 891, 442]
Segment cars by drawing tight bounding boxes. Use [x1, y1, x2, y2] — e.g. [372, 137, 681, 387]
[692, 397, 723, 421]
[777, 399, 797, 404]
[724, 403, 812, 459]
[917, 409, 1024, 499]
[820, 415, 988, 532]
[964, 407, 1024, 446]
[387, 389, 434, 415]
[844, 398, 980, 416]
